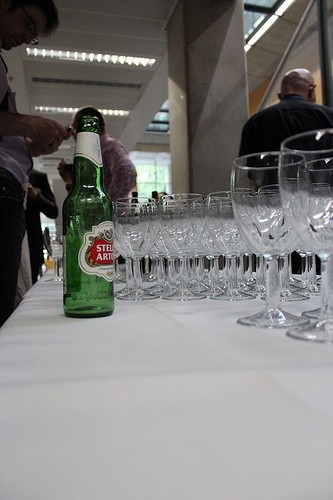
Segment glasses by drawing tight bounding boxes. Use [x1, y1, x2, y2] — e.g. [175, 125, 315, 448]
[19, 3, 40, 46]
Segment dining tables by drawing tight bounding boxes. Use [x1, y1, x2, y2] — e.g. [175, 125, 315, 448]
[0, 266, 333, 500]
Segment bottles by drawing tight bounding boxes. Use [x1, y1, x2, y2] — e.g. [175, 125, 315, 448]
[63, 116, 114, 318]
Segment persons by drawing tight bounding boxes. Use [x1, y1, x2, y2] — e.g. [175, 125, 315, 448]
[0, 0, 71, 327]
[57, 106, 137, 218]
[238, 69, 333, 276]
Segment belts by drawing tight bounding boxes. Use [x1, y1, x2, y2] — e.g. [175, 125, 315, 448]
[0, 168, 25, 202]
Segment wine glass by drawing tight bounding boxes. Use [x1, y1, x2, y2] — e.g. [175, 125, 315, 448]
[47, 226, 64, 282]
[111, 128, 333, 343]
